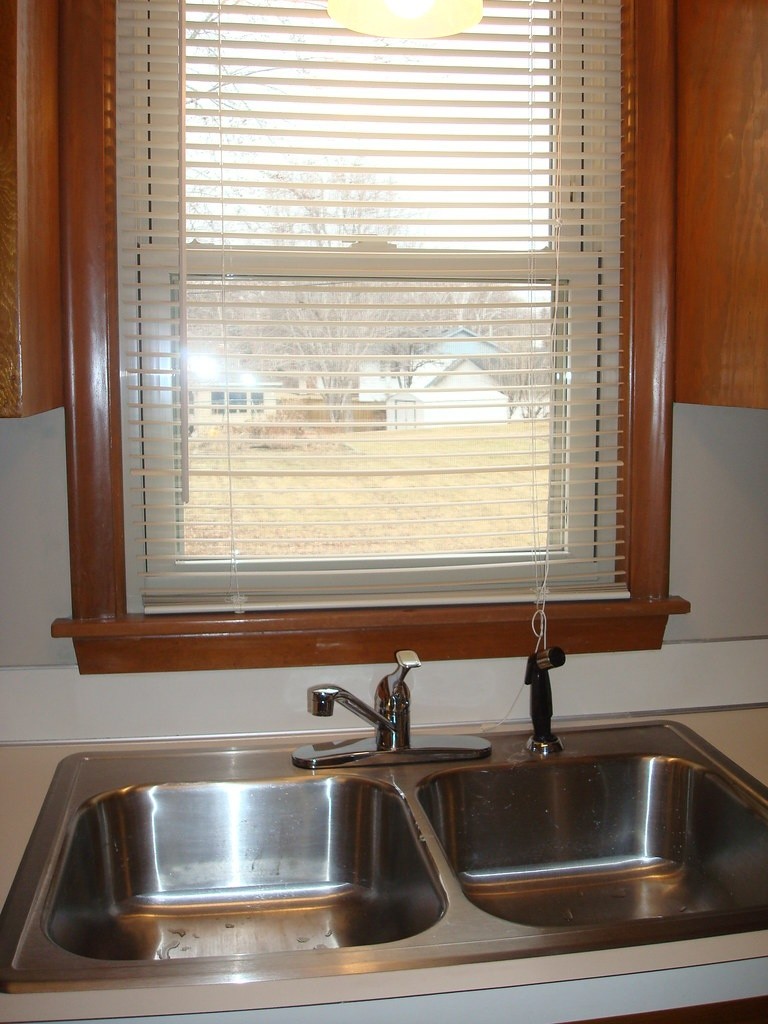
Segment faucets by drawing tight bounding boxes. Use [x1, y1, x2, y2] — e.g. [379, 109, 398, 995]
[292, 649, 493, 771]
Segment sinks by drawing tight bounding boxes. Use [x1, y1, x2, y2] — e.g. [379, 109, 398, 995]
[399, 720, 768, 964]
[0, 739, 461, 1024]
